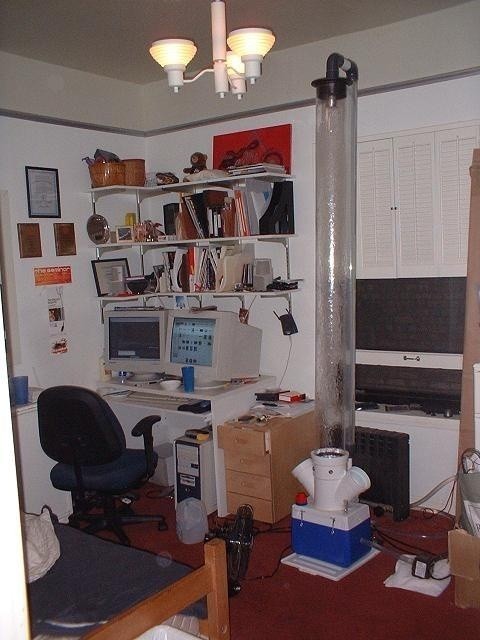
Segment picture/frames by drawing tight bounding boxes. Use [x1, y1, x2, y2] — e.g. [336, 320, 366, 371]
[90, 257, 133, 297]
[115, 224, 135, 244]
[25, 165, 62, 219]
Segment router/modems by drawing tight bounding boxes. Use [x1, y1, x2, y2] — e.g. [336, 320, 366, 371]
[273, 307, 298, 336]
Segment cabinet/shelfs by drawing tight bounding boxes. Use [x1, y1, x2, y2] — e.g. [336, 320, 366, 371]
[84, 171, 304, 326]
[216, 408, 320, 525]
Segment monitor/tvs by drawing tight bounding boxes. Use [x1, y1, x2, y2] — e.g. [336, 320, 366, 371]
[164, 309, 263, 389]
[104, 309, 169, 384]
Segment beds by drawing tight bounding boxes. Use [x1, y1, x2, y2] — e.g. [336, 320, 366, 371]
[26, 521, 232, 639]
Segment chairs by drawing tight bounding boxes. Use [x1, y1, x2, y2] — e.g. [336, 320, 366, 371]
[36, 384, 169, 546]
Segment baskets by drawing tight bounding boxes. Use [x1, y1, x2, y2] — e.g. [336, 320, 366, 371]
[122, 159, 145, 186]
[88, 163, 125, 188]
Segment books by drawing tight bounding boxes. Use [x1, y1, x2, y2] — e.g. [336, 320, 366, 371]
[153, 247, 221, 292]
[183, 193, 247, 239]
[279, 391, 306, 402]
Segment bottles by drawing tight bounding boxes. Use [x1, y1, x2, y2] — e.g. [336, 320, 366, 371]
[160, 273, 169, 293]
[118, 359, 128, 384]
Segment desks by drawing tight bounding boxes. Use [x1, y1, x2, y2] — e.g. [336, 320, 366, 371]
[94, 374, 277, 519]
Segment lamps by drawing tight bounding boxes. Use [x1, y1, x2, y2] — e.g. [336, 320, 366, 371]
[147, 0, 277, 101]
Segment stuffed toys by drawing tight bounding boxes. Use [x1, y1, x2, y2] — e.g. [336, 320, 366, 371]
[183, 152, 208, 175]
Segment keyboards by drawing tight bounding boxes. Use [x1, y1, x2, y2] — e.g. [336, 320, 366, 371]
[126, 391, 191, 405]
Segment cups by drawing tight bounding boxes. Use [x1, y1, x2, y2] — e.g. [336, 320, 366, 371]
[181, 366, 194, 391]
[10, 375, 29, 405]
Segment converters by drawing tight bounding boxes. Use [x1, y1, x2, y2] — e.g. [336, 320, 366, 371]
[413, 554, 433, 579]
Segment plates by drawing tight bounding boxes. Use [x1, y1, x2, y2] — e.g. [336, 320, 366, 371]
[87, 215, 109, 244]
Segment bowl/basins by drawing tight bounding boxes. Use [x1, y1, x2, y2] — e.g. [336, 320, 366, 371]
[126, 282, 148, 296]
[161, 381, 181, 391]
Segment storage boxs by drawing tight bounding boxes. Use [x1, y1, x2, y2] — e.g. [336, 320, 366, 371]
[447, 529, 480, 609]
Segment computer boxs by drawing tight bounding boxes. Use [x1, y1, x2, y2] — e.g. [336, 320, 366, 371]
[173, 424, 217, 516]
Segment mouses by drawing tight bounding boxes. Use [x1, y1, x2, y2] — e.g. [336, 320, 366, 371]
[200, 399, 210, 408]
